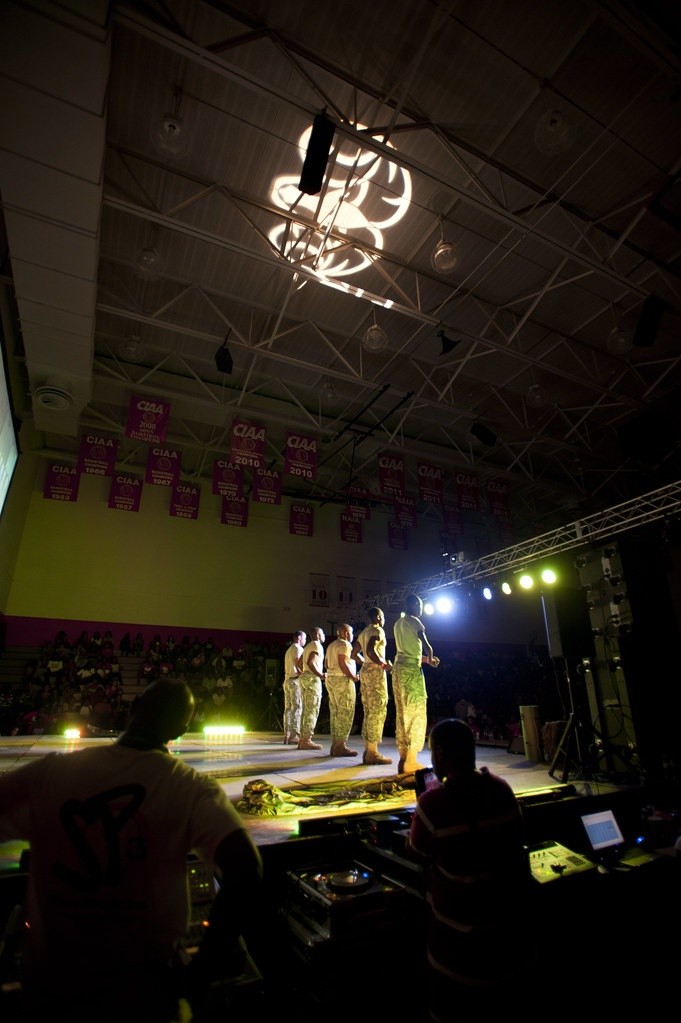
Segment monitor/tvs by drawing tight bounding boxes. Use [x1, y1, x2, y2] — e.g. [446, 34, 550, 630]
[581, 809, 625, 851]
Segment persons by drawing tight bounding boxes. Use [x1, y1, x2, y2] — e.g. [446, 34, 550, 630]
[297, 627, 326, 749]
[283, 631, 307, 745]
[351, 608, 393, 764]
[407, 719, 521, 1023]
[0, 678, 262, 1023]
[390, 595, 439, 774]
[0, 629, 266, 735]
[325, 624, 360, 757]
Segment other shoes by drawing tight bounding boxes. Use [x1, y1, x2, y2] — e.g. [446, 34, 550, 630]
[330, 744, 358, 757]
[363, 752, 392, 764]
[288, 738, 300, 744]
[398, 761, 424, 774]
[284, 736, 289, 744]
[297, 740, 323, 750]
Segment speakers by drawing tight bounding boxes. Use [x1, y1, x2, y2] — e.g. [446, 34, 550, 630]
[574, 540, 635, 638]
[261, 659, 279, 686]
[542, 588, 596, 664]
[594, 637, 681, 772]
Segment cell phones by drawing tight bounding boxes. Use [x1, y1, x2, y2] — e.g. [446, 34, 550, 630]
[423, 768, 439, 791]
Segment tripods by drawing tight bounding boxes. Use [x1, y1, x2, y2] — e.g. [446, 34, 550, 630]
[250, 686, 284, 732]
[547, 655, 642, 785]
